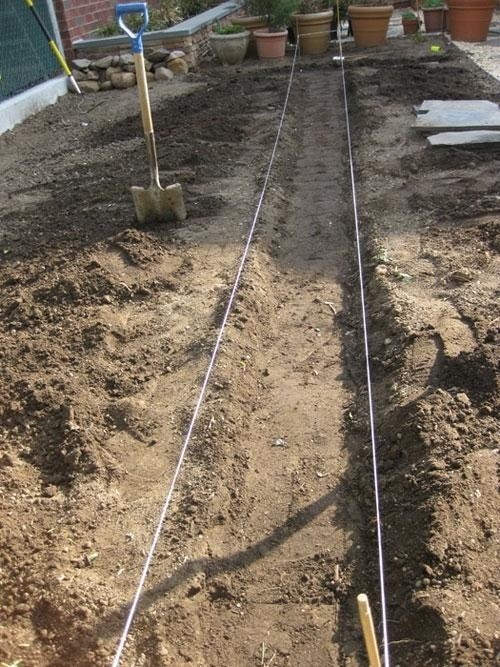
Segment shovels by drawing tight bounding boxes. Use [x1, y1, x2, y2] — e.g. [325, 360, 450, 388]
[115, 3, 187, 224]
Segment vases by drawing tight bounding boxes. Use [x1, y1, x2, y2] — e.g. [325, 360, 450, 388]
[447, 0, 497, 42]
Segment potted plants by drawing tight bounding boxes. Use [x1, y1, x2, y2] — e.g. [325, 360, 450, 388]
[209, 0, 449, 64]
